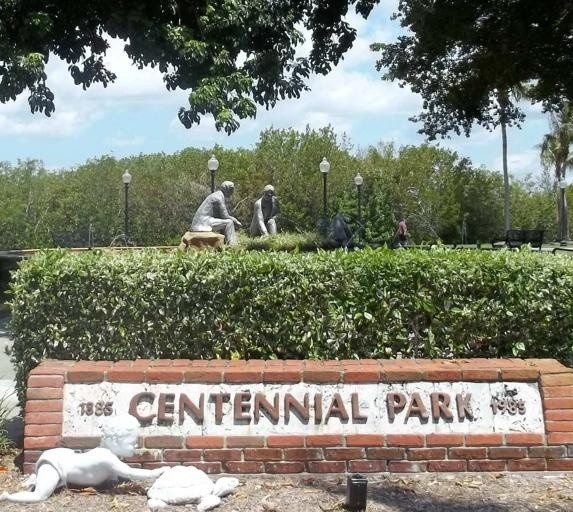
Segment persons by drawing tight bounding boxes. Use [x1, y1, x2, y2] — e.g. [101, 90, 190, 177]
[188, 179, 243, 246]
[0, 412, 172, 504]
[398, 217, 407, 242]
[249, 184, 282, 238]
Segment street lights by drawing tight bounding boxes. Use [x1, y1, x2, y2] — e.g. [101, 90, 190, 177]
[319, 156, 330, 211]
[207, 153, 220, 193]
[122, 169, 132, 235]
[354, 170, 364, 220]
[558, 178, 569, 242]
